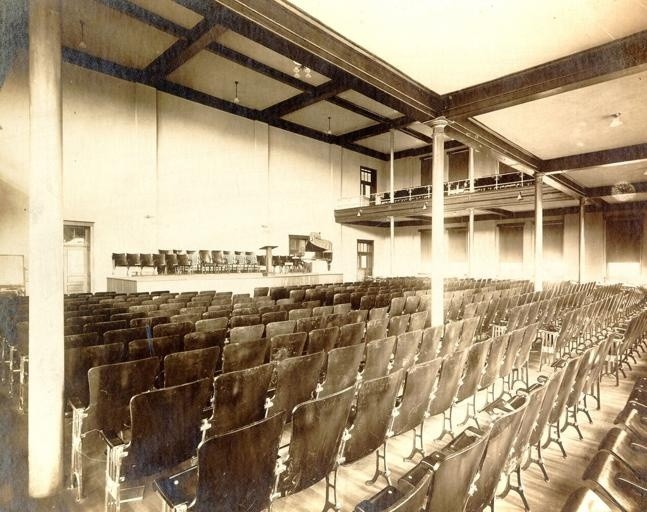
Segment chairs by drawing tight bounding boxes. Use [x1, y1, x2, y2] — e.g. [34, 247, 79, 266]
[110, 249, 311, 276]
[1, 278, 647, 512]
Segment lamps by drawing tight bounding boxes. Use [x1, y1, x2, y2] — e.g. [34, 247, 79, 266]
[608, 112, 624, 127]
[292, 60, 312, 79]
[422, 202, 428, 209]
[77, 17, 88, 49]
[328, 117, 331, 134]
[356, 209, 362, 217]
[233, 81, 240, 105]
[517, 192, 523, 200]
